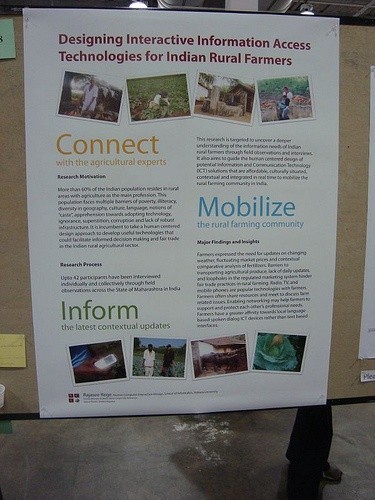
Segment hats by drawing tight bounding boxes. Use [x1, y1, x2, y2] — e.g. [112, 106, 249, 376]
[148, 344, 153, 346]
[166, 344, 171, 347]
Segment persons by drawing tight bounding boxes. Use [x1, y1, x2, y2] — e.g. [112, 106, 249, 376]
[277, 87, 293, 120]
[80, 75, 99, 119]
[142, 344, 156, 377]
[161, 344, 175, 377]
[284, 406, 344, 500]
[149, 91, 171, 108]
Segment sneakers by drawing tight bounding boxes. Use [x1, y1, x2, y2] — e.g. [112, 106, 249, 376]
[322, 463, 342, 481]
